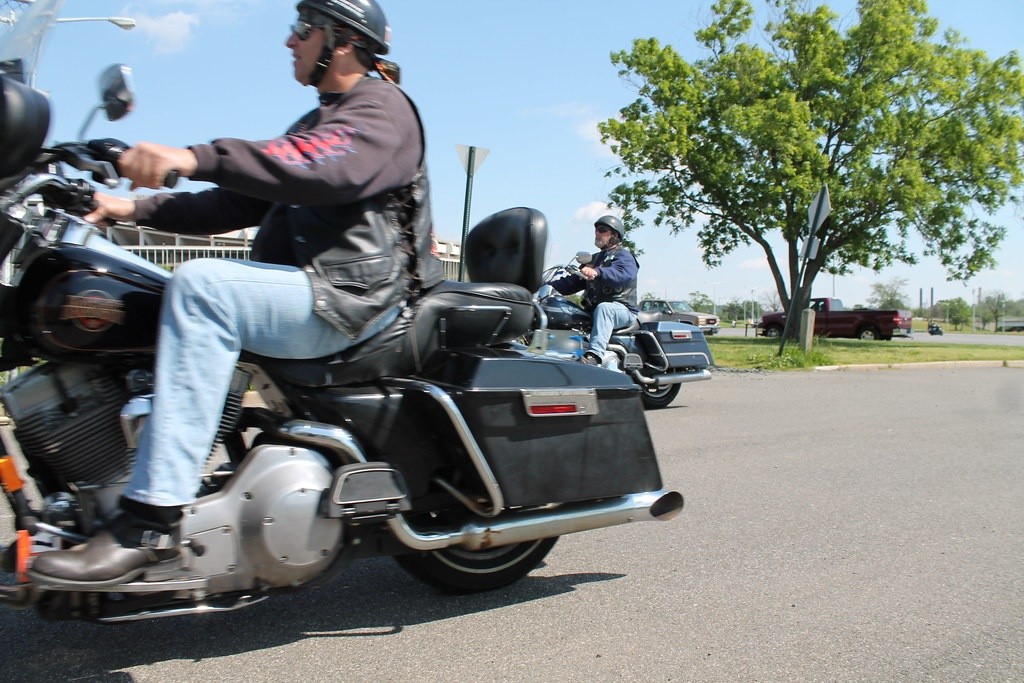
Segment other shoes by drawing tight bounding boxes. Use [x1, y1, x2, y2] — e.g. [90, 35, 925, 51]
[580, 352, 602, 367]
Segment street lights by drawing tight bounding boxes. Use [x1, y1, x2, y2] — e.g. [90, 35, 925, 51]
[23, 19, 136, 87]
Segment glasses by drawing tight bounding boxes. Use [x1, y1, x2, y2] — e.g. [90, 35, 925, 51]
[594, 226, 611, 233]
[291, 21, 342, 41]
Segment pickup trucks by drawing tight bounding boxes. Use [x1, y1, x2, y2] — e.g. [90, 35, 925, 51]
[753, 296, 914, 343]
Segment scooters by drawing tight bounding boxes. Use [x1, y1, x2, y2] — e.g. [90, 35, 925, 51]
[927, 319, 945, 336]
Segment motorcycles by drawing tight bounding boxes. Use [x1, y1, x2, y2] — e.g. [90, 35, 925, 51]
[0, 58, 684, 626]
[515, 248, 715, 409]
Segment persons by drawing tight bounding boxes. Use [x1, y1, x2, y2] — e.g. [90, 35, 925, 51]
[542, 215, 639, 364]
[22, 1, 445, 592]
[928, 320, 936, 333]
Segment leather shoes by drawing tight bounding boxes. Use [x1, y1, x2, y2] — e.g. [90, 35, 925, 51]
[25, 509, 184, 588]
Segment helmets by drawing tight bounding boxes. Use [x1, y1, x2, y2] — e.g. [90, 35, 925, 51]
[594, 215, 624, 242]
[297, 0, 392, 55]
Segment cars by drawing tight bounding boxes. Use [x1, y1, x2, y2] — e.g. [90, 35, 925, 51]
[636, 298, 723, 337]
